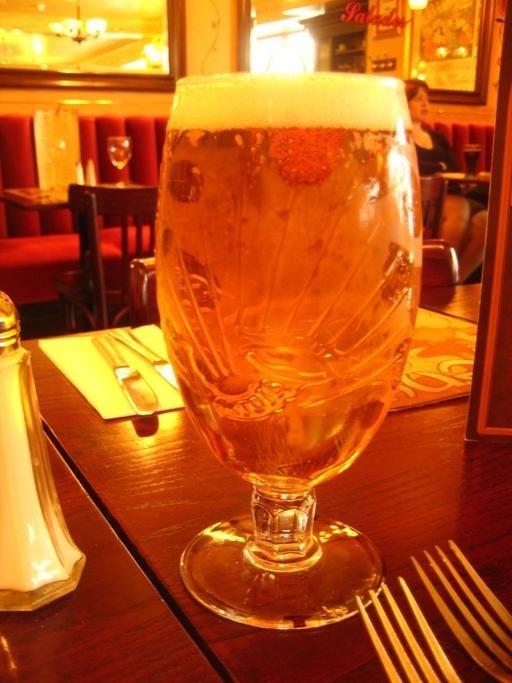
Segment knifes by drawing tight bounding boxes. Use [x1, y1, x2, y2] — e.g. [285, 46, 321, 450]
[95, 329, 180, 418]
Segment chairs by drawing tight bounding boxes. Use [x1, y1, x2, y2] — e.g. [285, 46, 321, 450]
[0, 171, 511, 683]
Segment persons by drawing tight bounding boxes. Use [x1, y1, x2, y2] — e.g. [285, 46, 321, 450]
[400, 76, 461, 195]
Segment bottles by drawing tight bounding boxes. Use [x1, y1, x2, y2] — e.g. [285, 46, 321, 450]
[76, 160, 96, 190]
[0, 285, 88, 613]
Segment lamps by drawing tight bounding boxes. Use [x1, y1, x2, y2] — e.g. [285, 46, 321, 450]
[48, 0, 108, 45]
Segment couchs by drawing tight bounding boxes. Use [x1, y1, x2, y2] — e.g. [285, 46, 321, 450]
[0, 116, 172, 307]
[415, 122, 498, 203]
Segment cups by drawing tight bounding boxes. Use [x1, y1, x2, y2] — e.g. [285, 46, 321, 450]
[464, 144, 481, 176]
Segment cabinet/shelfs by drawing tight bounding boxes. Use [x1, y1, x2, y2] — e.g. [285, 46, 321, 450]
[299, 9, 367, 73]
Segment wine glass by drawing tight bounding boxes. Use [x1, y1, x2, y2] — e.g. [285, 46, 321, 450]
[153, 70, 423, 631]
[108, 135, 132, 187]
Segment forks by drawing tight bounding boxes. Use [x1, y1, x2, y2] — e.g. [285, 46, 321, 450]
[355, 540, 512, 682]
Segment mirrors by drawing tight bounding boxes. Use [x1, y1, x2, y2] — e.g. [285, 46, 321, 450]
[237, 0, 494, 106]
[0, 0, 188, 93]
